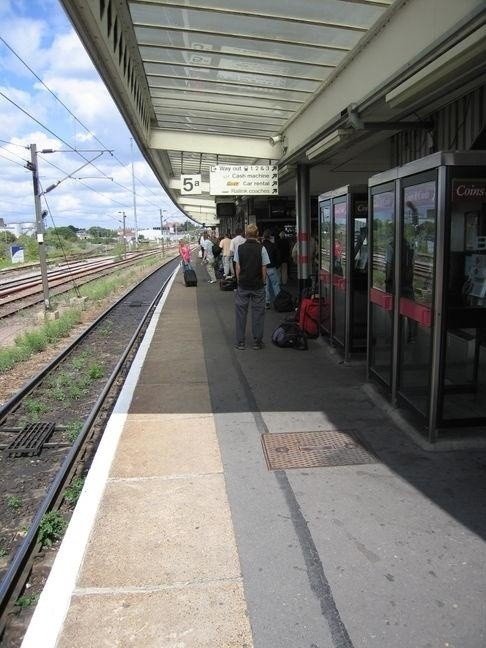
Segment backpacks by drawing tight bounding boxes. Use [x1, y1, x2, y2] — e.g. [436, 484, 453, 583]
[212, 243, 222, 258]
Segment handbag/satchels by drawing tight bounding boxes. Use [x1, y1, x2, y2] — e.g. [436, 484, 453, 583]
[272, 288, 296, 314]
[220, 278, 238, 291]
[271, 321, 309, 350]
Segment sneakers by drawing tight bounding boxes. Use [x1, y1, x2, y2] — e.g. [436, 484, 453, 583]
[207, 279, 217, 284]
[252, 341, 265, 350]
[232, 340, 246, 350]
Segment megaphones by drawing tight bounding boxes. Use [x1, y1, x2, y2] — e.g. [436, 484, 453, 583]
[269, 134, 283, 145]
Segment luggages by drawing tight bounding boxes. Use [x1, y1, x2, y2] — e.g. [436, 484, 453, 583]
[299, 297, 322, 339]
[184, 270, 198, 287]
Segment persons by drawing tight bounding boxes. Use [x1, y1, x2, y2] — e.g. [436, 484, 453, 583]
[198, 228, 319, 310]
[178, 238, 192, 285]
[334, 238, 344, 277]
[231, 223, 271, 351]
[385, 223, 417, 343]
